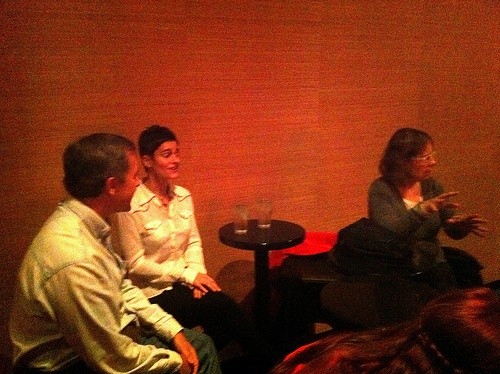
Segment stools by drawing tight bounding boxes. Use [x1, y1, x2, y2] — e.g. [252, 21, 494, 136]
[280, 252, 346, 353]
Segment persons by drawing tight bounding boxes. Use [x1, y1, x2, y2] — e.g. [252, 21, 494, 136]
[275, 290, 500, 374]
[9, 132, 223, 374]
[366, 128, 489, 287]
[110, 127, 284, 363]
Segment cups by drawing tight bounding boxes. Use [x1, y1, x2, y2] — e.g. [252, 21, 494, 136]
[233, 205, 248, 235]
[256, 200, 272, 229]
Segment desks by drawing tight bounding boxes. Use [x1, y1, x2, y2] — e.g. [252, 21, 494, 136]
[220, 219, 305, 340]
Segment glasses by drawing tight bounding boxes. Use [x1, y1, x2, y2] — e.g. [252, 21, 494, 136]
[410, 150, 438, 162]
